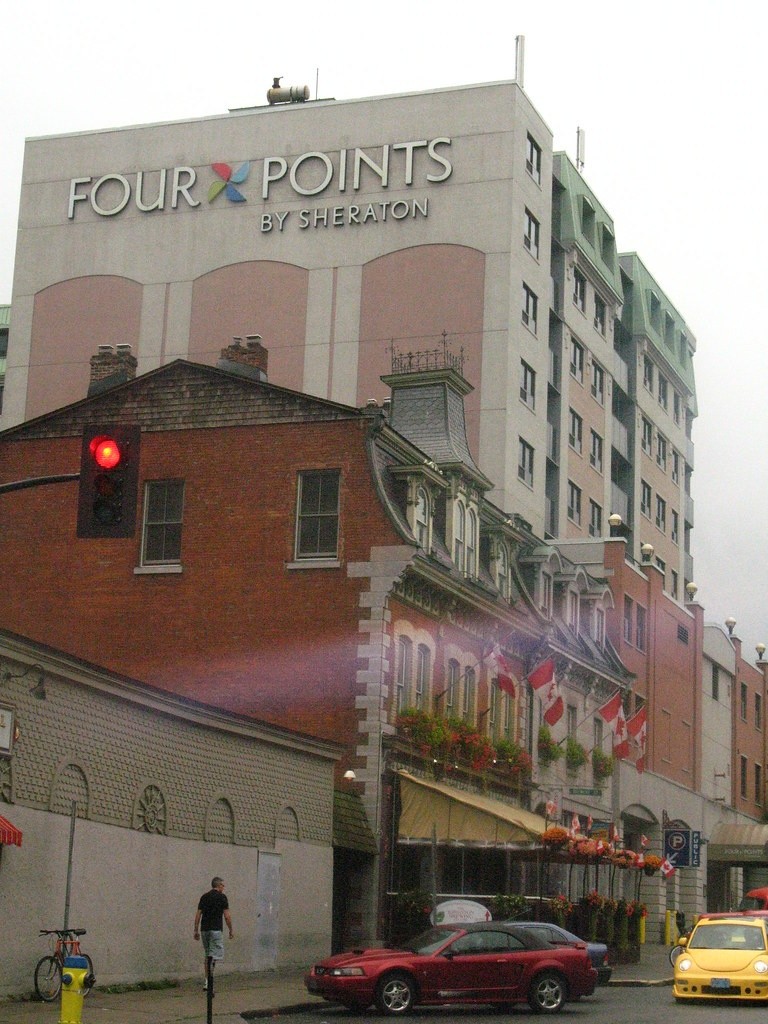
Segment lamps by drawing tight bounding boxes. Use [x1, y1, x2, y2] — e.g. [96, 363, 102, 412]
[4, 663, 46, 700]
[343, 771, 356, 781]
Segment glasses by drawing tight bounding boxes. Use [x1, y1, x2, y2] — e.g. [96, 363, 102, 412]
[221, 883, 225, 887]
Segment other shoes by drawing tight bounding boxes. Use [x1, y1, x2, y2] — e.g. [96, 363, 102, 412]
[203, 978, 214, 996]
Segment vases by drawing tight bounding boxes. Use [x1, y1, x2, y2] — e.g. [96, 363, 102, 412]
[602, 942, 641, 965]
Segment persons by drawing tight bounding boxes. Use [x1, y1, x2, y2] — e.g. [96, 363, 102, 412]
[194, 877, 234, 989]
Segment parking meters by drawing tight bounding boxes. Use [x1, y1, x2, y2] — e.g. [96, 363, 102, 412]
[676, 910, 685, 937]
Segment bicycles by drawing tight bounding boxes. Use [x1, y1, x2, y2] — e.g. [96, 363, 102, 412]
[669, 945, 687, 968]
[34, 928, 94, 1002]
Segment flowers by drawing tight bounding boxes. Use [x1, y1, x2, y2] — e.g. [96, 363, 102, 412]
[542, 827, 661, 872]
[394, 706, 531, 789]
[389, 884, 643, 937]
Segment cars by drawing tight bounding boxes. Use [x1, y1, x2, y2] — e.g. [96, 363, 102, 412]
[305, 922, 598, 1017]
[503, 923, 612, 1003]
[672, 917, 768, 1005]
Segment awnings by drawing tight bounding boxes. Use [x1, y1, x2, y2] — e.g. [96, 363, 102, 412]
[396, 763, 644, 865]
[0, 816, 22, 847]
[708, 819, 768, 867]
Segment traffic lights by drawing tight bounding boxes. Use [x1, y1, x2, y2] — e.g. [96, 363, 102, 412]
[77, 424, 140, 539]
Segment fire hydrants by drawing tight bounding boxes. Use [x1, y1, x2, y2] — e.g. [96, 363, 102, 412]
[58, 955, 96, 1024]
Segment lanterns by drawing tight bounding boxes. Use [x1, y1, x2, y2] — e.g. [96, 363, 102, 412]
[95, 441, 121, 468]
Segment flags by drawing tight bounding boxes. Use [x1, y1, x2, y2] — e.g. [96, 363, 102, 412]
[546, 797, 559, 814]
[641, 834, 648, 845]
[626, 705, 646, 774]
[483, 619, 515, 699]
[657, 856, 675, 878]
[635, 851, 645, 867]
[588, 814, 594, 830]
[570, 813, 580, 835]
[614, 825, 620, 840]
[598, 690, 631, 760]
[593, 838, 605, 855]
[527, 659, 563, 725]
